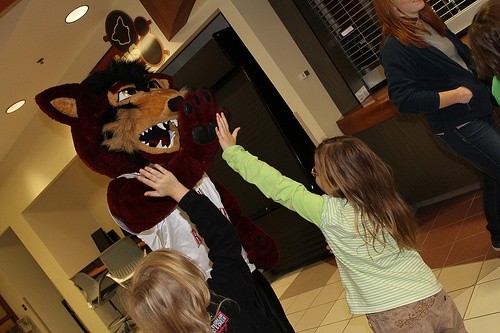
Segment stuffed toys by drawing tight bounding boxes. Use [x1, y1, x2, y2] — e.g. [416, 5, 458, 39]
[34, 59, 295, 333]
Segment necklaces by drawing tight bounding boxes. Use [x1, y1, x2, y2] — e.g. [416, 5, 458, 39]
[419, 18, 427, 31]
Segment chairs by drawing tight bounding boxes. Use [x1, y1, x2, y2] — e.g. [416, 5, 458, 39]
[68, 270, 137, 333]
[96, 236, 148, 289]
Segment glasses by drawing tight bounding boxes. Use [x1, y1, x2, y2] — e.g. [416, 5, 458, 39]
[311, 166, 319, 176]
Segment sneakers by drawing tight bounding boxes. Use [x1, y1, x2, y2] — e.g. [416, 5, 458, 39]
[490, 234, 500, 251]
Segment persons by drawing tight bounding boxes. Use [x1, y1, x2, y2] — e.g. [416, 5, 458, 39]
[469, 0, 500, 105]
[121, 164, 289, 333]
[215, 112, 468, 333]
[374, 0, 500, 252]
